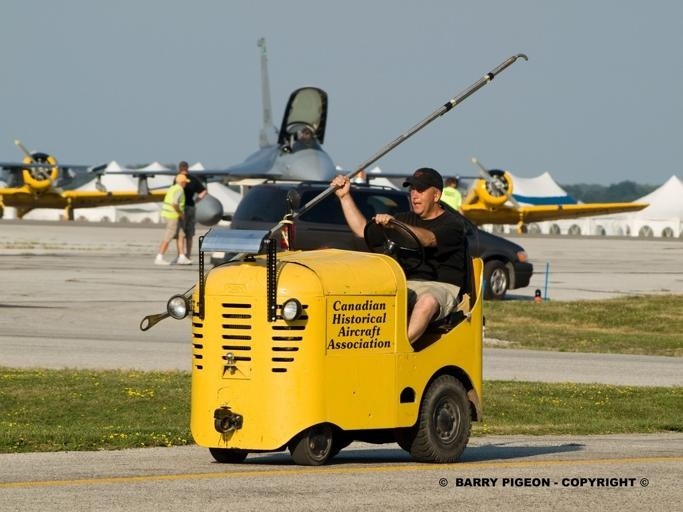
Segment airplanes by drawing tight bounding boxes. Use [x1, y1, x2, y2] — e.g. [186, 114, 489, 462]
[59, 33, 486, 230]
[438, 156, 650, 234]
[0, 137, 165, 220]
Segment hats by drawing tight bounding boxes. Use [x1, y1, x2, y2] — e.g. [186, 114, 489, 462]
[402, 167, 443, 193]
[176, 173, 191, 183]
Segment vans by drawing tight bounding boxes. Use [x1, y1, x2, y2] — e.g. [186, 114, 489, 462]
[204, 174, 535, 302]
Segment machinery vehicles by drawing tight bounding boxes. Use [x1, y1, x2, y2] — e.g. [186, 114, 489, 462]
[137, 225, 489, 469]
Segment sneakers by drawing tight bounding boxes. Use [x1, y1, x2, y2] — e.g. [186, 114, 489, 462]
[154, 256, 171, 265]
[177, 256, 193, 265]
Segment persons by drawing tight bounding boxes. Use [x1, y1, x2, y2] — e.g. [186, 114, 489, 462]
[439, 177, 464, 216]
[172, 161, 208, 261]
[329, 167, 468, 345]
[153, 173, 193, 266]
